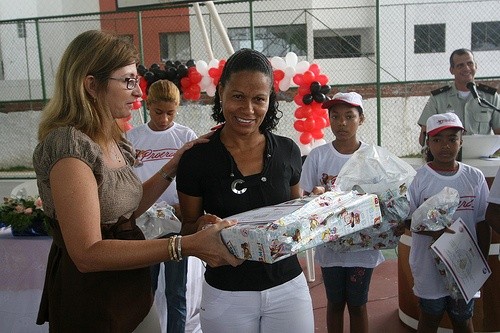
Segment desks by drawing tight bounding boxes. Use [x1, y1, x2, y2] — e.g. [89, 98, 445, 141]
[0, 227, 161, 333]
[398, 157, 500, 333]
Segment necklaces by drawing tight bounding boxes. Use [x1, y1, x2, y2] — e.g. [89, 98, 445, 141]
[110, 147, 121, 163]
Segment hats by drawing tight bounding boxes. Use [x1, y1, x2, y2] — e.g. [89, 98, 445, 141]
[426, 112, 467, 140]
[321, 92, 364, 114]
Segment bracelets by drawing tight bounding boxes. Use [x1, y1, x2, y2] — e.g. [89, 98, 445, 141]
[167, 234, 183, 265]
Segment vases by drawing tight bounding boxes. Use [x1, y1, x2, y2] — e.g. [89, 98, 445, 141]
[36, 225, 48, 236]
[10, 224, 32, 237]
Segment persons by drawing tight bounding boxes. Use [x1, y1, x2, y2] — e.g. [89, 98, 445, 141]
[408, 114, 490, 333]
[33, 30, 246, 333]
[176, 47, 315, 333]
[419, 49, 500, 154]
[124, 80, 198, 333]
[485, 167, 500, 235]
[300, 93, 384, 333]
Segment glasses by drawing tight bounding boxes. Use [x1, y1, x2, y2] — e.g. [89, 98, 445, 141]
[93, 74, 142, 90]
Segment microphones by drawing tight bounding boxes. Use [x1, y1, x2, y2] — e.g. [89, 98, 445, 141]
[466, 82, 481, 104]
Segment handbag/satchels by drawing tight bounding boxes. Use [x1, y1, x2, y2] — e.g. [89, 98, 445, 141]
[326, 143, 417, 254]
[135, 201, 182, 240]
[410, 186, 463, 301]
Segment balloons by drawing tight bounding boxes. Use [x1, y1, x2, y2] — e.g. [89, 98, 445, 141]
[111, 52, 331, 143]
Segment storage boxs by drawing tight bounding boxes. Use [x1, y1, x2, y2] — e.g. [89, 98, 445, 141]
[202, 191, 382, 264]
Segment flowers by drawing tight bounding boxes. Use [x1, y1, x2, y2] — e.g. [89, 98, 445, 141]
[34, 197, 53, 235]
[0, 197, 37, 231]
